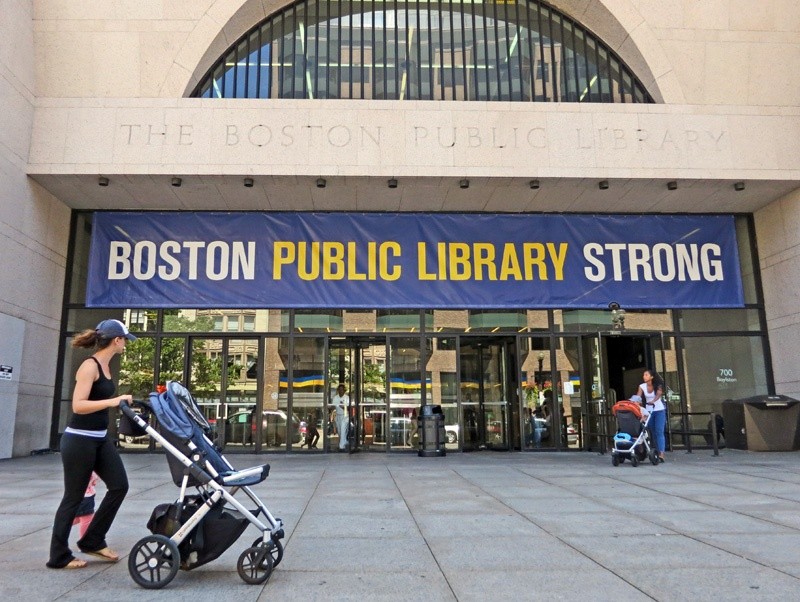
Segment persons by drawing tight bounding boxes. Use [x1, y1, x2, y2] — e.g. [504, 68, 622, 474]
[300, 408, 338, 450]
[331, 383, 359, 450]
[638, 369, 667, 464]
[407, 405, 420, 447]
[541, 389, 564, 446]
[463, 394, 479, 446]
[45, 318, 137, 569]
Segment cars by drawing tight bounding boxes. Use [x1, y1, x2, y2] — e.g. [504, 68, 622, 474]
[389, 416, 549, 447]
[567, 423, 580, 445]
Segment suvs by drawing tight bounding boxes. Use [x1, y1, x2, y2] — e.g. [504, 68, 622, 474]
[206, 408, 303, 448]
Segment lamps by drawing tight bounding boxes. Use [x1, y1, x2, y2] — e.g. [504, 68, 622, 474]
[172, 179, 181, 187]
[530, 180, 540, 189]
[599, 181, 609, 189]
[459, 180, 469, 188]
[611, 308, 627, 332]
[243, 178, 254, 187]
[316, 179, 326, 187]
[387, 180, 398, 188]
[667, 181, 678, 190]
[734, 183, 745, 190]
[99, 179, 109, 186]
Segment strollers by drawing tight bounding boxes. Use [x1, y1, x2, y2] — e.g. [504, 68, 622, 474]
[118, 380, 285, 590]
[610, 399, 660, 467]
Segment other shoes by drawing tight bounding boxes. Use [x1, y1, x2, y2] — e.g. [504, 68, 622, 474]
[659, 455, 664, 462]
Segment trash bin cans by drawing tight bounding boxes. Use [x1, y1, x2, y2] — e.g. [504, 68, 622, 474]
[417, 405, 446, 457]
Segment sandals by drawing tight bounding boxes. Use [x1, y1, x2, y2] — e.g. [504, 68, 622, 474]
[64, 558, 87, 569]
[88, 546, 119, 561]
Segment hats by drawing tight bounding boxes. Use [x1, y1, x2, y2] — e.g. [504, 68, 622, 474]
[96, 319, 136, 342]
